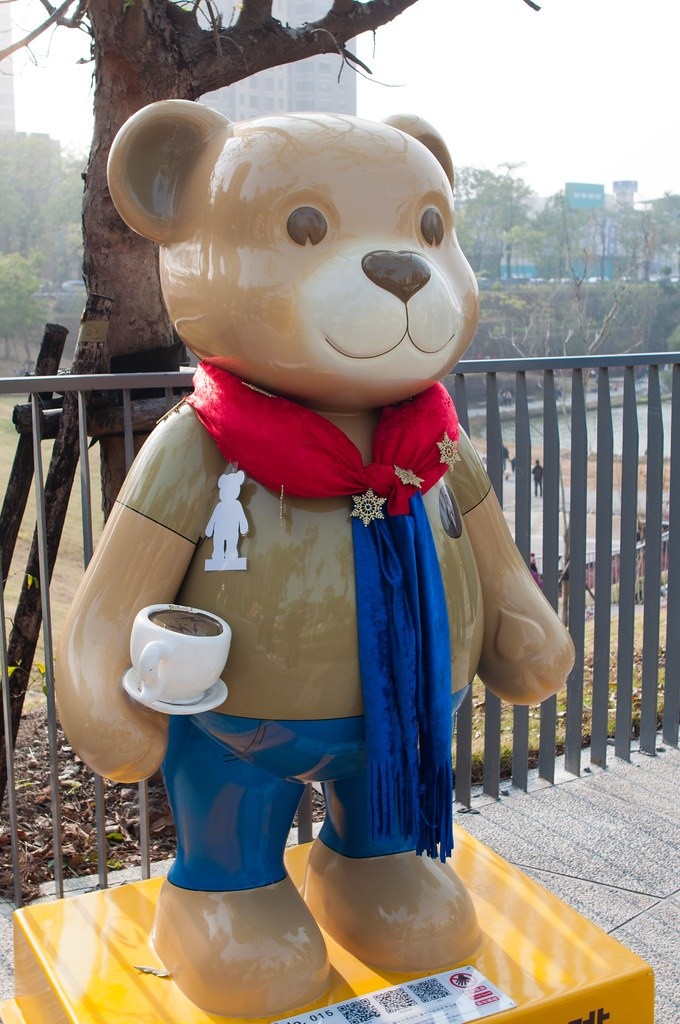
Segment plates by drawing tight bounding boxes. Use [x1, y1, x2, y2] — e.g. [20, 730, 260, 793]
[121, 666, 228, 714]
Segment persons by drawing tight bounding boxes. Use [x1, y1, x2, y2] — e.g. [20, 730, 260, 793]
[532, 460, 544, 498]
[501, 443, 517, 476]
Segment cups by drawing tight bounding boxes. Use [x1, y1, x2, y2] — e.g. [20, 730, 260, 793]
[129, 604, 231, 705]
